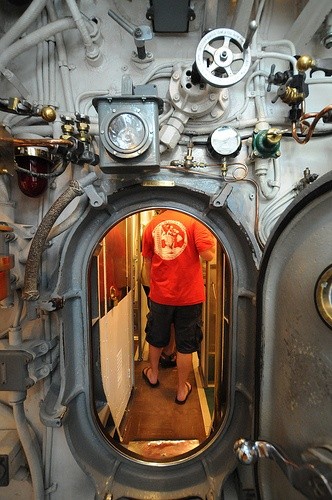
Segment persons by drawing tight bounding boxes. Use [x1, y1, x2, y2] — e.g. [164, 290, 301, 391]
[139, 209, 219, 404]
[137, 207, 179, 369]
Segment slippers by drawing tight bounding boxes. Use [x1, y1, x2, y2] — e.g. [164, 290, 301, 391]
[142, 366, 160, 387]
[175, 380, 192, 404]
